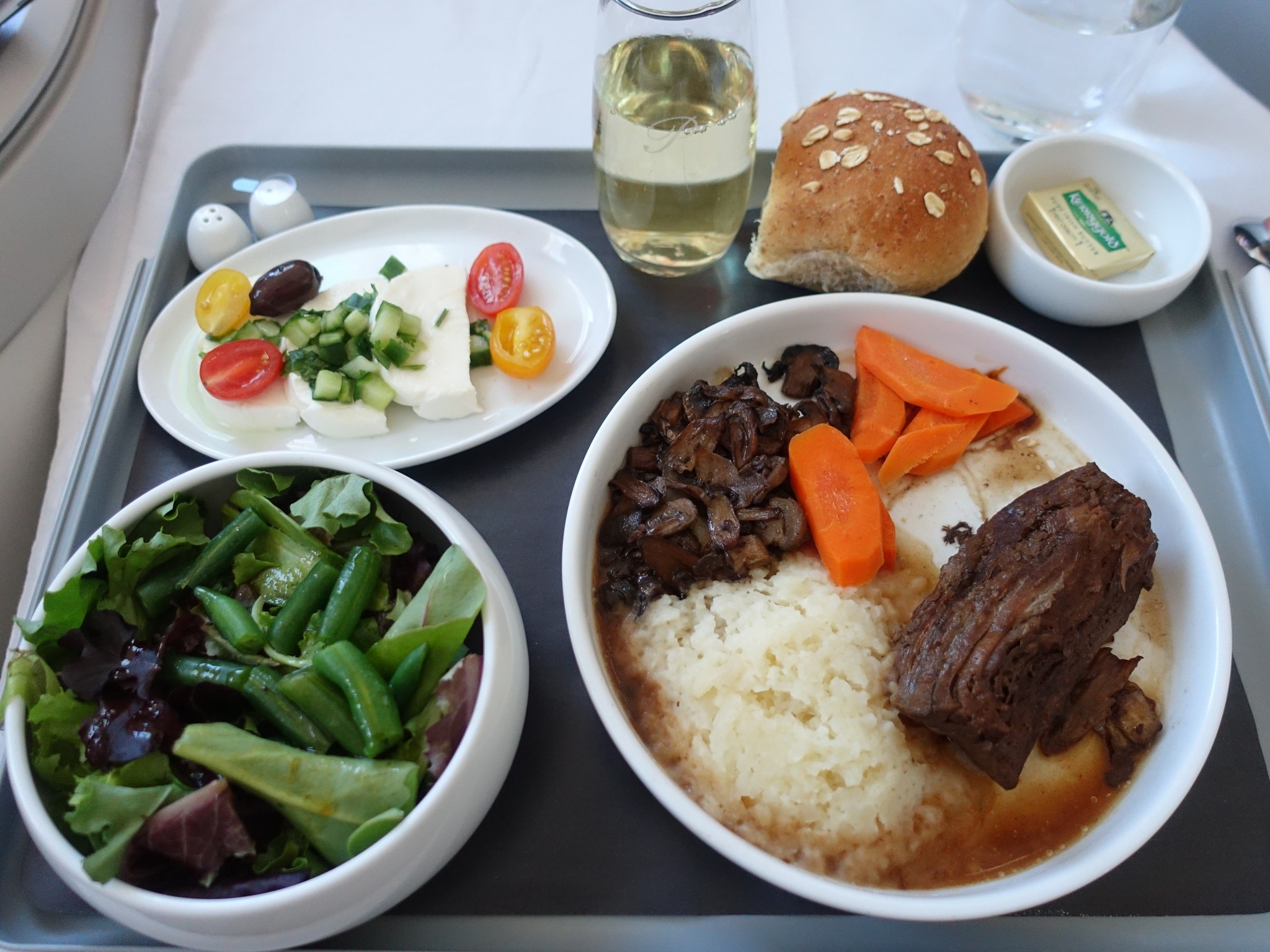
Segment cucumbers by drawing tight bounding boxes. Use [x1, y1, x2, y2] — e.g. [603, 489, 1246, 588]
[225, 288, 422, 407]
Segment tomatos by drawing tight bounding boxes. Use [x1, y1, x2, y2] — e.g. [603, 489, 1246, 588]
[466, 242, 555, 380]
[195, 259, 323, 403]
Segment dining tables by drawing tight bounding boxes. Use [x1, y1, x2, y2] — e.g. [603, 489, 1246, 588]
[2, 0, 1270, 951]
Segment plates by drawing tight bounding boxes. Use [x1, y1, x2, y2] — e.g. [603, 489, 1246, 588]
[136, 202, 620, 470]
[560, 290, 1236, 924]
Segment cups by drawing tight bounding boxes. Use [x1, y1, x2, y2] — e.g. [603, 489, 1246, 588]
[593, 0, 764, 276]
[954, 0, 1181, 144]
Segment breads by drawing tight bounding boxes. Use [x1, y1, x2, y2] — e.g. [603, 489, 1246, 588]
[744, 91, 988, 296]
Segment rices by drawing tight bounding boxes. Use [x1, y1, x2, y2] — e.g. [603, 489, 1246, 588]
[624, 345, 1171, 889]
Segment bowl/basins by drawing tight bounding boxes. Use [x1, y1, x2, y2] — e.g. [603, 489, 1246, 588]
[989, 134, 1213, 326]
[1, 454, 533, 952]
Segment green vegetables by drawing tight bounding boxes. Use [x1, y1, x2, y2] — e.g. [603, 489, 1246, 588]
[4, 464, 489, 901]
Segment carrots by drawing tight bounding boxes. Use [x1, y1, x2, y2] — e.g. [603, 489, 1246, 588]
[787, 323, 1037, 587]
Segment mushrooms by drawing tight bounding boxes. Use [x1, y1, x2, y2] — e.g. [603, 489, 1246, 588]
[596, 340, 858, 603]
